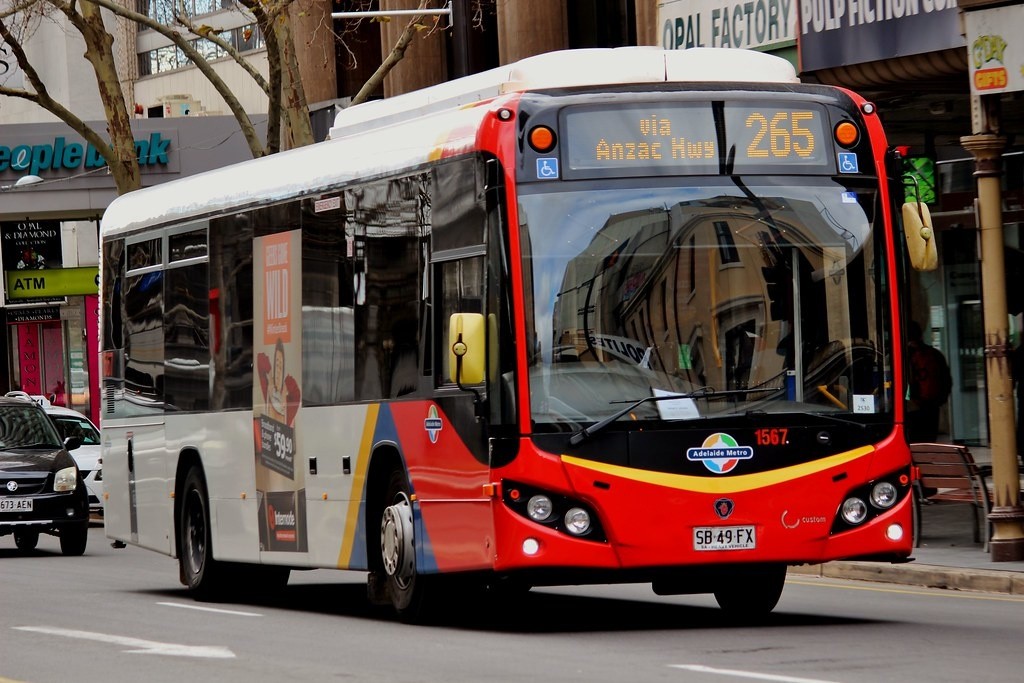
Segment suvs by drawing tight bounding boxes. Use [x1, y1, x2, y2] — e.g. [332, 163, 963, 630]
[0, 391, 89, 556]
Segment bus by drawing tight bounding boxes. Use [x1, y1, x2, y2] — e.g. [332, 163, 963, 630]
[96, 46, 938, 626]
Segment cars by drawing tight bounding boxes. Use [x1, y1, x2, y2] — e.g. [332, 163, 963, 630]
[40, 404, 105, 517]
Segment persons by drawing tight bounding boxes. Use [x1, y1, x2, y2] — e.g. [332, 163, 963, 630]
[256, 337, 300, 426]
[909, 324, 953, 500]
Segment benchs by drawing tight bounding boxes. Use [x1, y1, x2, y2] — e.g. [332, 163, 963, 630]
[908, 443, 992, 554]
